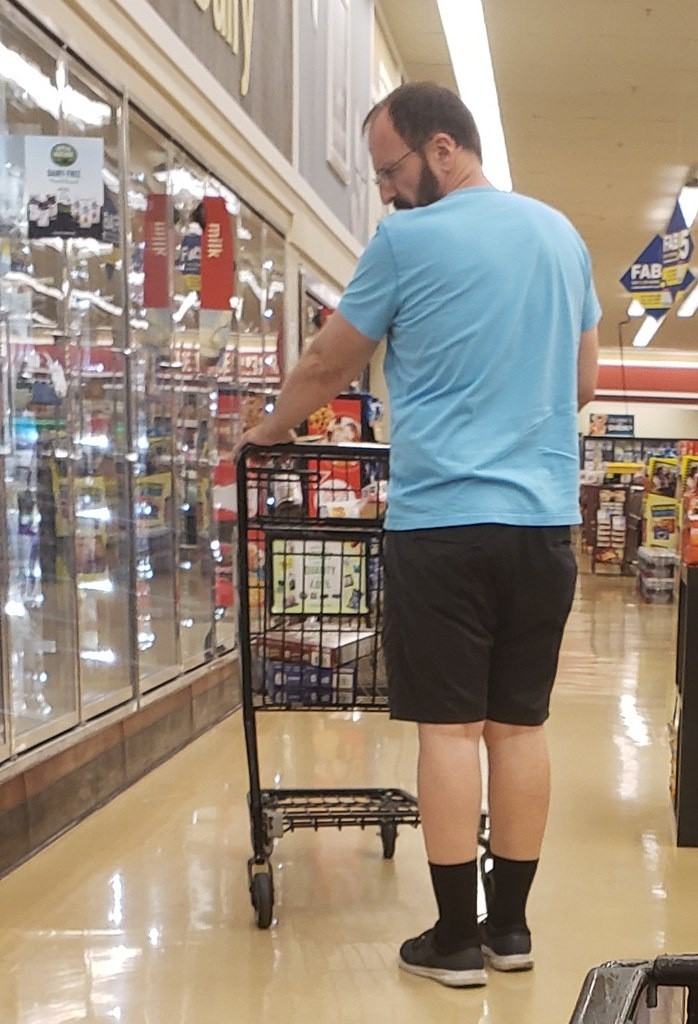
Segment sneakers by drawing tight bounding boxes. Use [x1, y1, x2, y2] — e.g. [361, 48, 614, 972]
[397, 926, 489, 986]
[476, 913, 533, 971]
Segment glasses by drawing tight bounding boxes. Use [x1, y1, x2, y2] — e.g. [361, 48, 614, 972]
[370, 149, 417, 187]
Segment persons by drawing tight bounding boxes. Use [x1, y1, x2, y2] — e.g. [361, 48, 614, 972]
[230, 81, 605, 987]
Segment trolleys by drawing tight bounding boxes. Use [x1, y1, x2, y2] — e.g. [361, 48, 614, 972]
[232, 442, 491, 929]
